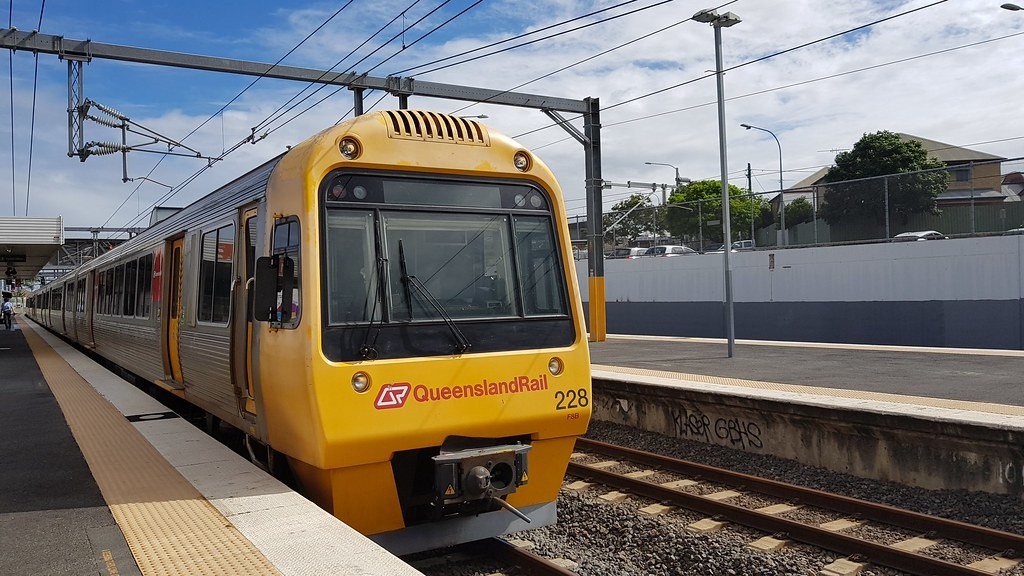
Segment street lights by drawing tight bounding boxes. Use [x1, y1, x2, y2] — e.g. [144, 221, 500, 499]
[644, 161, 679, 193]
[690, 7, 742, 357]
[739, 123, 786, 250]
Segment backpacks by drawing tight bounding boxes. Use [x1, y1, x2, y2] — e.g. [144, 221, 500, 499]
[2, 303, 12, 314]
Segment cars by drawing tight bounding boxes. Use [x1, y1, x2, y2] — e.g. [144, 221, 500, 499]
[606, 246, 699, 259]
[893, 230, 949, 241]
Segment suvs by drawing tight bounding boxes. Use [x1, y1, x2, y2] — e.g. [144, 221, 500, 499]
[698, 240, 752, 252]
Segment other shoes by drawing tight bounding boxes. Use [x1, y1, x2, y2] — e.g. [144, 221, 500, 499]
[5, 326, 11, 331]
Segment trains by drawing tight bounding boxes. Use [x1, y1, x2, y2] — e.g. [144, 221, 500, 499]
[26, 110, 592, 557]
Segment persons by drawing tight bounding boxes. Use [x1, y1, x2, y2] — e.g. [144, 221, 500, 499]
[0, 297, 16, 330]
